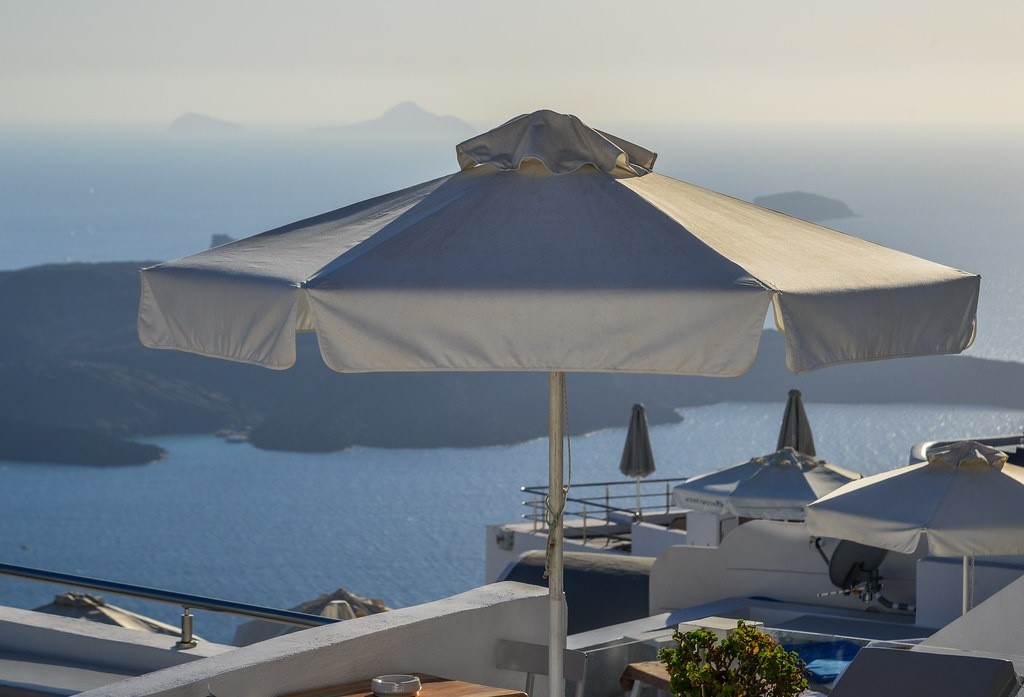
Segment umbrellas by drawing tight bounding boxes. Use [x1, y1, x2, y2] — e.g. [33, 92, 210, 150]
[777, 388, 816, 457]
[804, 439, 1024, 614]
[136, 108, 981, 697]
[620, 400, 656, 512]
[231, 588, 391, 647]
[670, 446, 865, 518]
[31, 591, 205, 640]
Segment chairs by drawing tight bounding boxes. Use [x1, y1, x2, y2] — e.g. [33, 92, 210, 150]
[493, 638, 587, 697]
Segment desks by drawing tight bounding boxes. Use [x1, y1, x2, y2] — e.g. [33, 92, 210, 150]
[277, 671, 529, 697]
